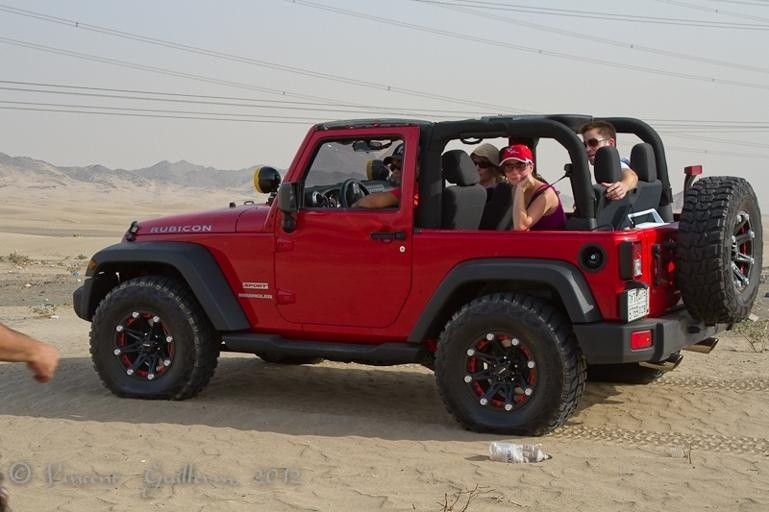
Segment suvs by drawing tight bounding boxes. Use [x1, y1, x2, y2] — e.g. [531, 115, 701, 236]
[73, 114, 763, 436]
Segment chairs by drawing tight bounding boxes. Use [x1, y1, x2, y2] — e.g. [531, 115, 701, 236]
[442, 150, 487, 229]
[482, 146, 513, 230]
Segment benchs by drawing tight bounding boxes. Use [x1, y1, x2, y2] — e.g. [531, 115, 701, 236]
[591, 143, 663, 230]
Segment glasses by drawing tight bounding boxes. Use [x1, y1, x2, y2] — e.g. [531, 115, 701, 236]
[584, 138, 610, 148]
[473, 160, 494, 168]
[504, 161, 530, 172]
[390, 165, 400, 172]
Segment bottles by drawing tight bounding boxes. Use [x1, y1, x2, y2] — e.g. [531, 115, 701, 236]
[487, 441, 550, 464]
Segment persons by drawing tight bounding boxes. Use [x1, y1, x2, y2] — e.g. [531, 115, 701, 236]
[348, 141, 424, 209]
[580, 119, 640, 201]
[468, 142, 508, 189]
[498, 143, 568, 232]
[1, 322, 62, 385]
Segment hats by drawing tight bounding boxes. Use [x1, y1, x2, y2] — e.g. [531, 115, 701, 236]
[469, 143, 500, 167]
[383, 143, 405, 166]
[498, 143, 535, 168]
